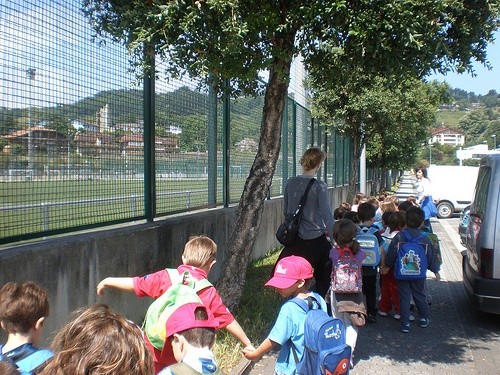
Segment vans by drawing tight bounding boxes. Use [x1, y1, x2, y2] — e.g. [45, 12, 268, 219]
[460, 152, 500, 316]
[426, 164, 479, 219]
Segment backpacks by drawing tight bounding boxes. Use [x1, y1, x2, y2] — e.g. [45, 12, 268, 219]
[140, 268, 213, 352]
[353, 225, 381, 266]
[330, 247, 363, 294]
[287, 293, 352, 375]
[393, 230, 428, 281]
[0, 349, 54, 375]
[421, 231, 442, 265]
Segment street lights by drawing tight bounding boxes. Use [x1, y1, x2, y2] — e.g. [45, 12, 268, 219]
[26, 67, 36, 170]
[428, 145, 433, 166]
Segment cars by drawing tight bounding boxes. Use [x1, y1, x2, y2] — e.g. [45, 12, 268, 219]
[457, 204, 471, 248]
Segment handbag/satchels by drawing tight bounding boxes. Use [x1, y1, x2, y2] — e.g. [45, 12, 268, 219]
[276, 213, 299, 247]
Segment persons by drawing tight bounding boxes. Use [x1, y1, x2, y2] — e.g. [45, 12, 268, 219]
[329, 220, 366, 369]
[284, 149, 335, 314]
[39, 303, 153, 375]
[333, 187, 419, 320]
[416, 166, 437, 232]
[381, 205, 440, 333]
[242, 256, 327, 375]
[97, 237, 255, 375]
[157, 302, 225, 375]
[0, 281, 54, 375]
[355, 202, 385, 321]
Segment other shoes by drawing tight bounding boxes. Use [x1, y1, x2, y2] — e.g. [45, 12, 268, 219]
[367, 312, 377, 322]
[420, 318, 430, 328]
[394, 314, 400, 320]
[409, 315, 416, 320]
[400, 323, 410, 333]
[378, 310, 388, 317]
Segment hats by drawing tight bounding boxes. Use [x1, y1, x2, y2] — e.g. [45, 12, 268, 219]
[264, 255, 313, 289]
[160, 302, 220, 358]
